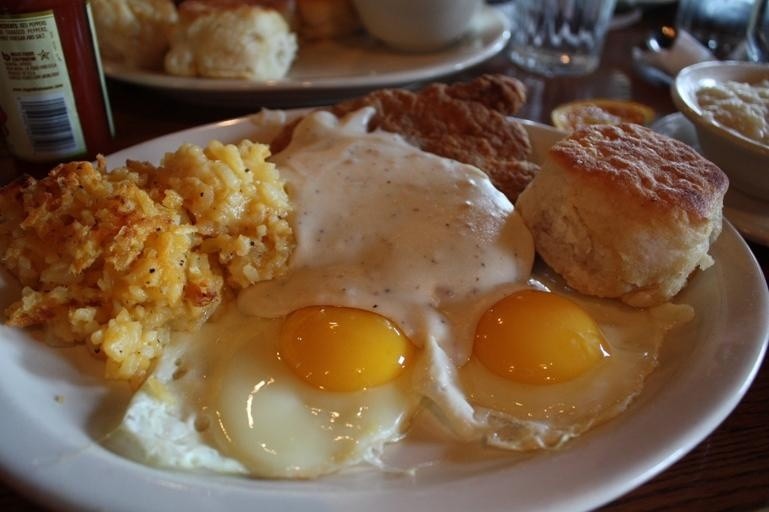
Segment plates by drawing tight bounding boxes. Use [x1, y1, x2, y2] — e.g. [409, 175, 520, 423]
[101, 6, 515, 94]
[0, 102, 769, 512]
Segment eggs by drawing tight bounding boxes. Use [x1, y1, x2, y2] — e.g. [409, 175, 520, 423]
[429, 276, 695, 456]
[220, 305, 424, 478]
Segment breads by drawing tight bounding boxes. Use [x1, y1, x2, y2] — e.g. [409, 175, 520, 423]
[514, 122, 728, 307]
[162, 8, 296, 84]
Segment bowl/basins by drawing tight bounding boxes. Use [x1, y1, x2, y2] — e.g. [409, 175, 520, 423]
[670, 57, 769, 200]
